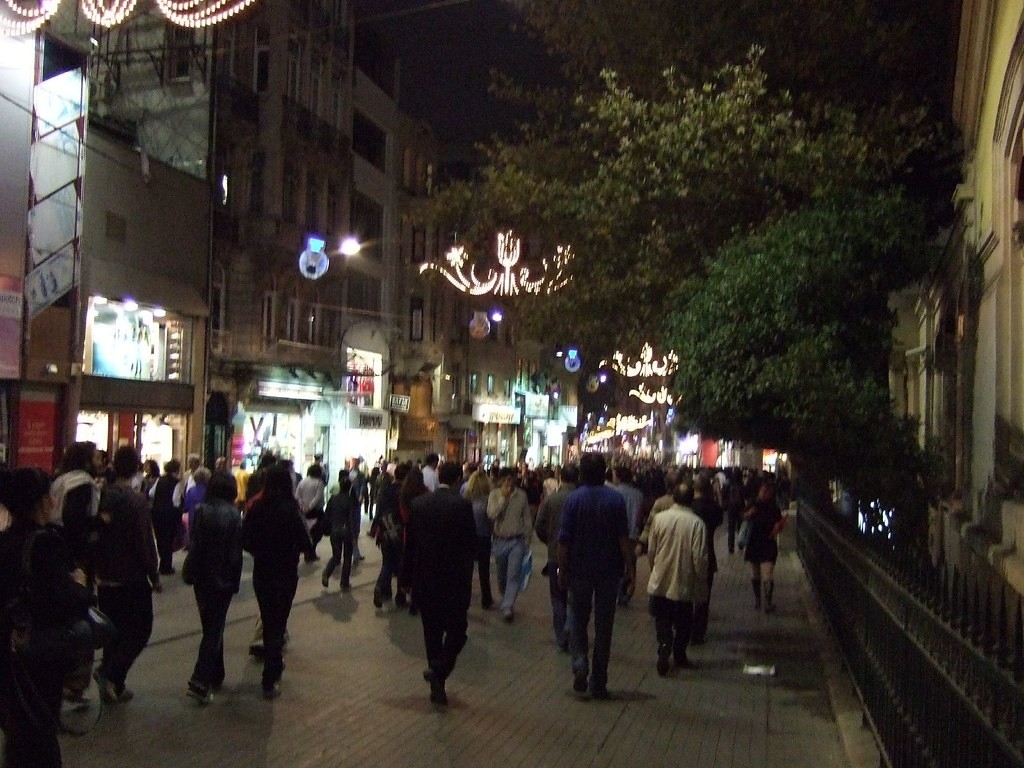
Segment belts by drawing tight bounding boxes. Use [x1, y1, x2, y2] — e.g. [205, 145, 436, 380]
[493, 533, 520, 540]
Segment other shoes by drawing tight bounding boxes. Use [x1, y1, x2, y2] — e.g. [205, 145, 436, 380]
[187, 688, 214, 703]
[423, 667, 445, 693]
[119, 687, 133, 700]
[503, 609, 514, 619]
[265, 684, 281, 698]
[676, 656, 689, 665]
[593, 687, 607, 696]
[658, 643, 670, 675]
[341, 583, 351, 591]
[483, 600, 495, 609]
[248, 647, 265, 657]
[321, 570, 329, 587]
[91, 670, 117, 705]
[573, 670, 587, 692]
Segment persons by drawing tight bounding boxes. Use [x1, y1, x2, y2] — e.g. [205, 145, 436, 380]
[646, 482, 709, 675]
[735, 478, 789, 614]
[0, 443, 793, 768]
[148, 458, 185, 574]
[557, 451, 630, 698]
[93, 447, 164, 705]
[486, 467, 532, 624]
[181, 472, 242, 706]
[242, 464, 311, 700]
[401, 461, 491, 704]
[322, 478, 360, 593]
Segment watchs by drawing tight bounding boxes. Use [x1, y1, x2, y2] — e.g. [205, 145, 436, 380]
[557, 568, 569, 574]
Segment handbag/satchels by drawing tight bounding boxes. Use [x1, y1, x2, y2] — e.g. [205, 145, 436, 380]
[6, 528, 113, 685]
[737, 518, 751, 547]
[519, 548, 533, 592]
[183, 509, 204, 584]
[321, 501, 333, 536]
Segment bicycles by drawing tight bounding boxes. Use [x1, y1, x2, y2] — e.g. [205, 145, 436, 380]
[58, 666, 103, 737]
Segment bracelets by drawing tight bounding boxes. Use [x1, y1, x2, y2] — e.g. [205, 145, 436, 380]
[638, 541, 643, 545]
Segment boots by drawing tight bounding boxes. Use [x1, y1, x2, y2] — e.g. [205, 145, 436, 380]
[763, 581, 774, 612]
[752, 577, 761, 606]
